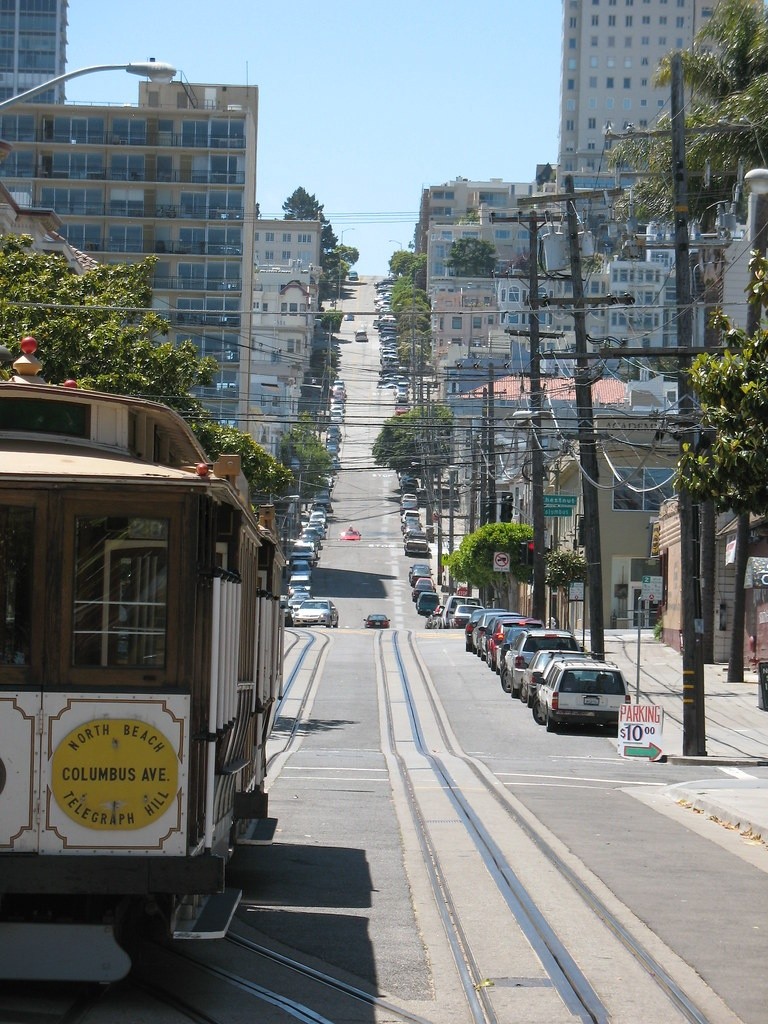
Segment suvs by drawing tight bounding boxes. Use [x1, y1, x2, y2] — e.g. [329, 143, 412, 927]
[531, 657, 632, 733]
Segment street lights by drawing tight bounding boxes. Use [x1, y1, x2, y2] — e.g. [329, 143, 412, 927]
[339, 227, 356, 301]
[389, 240, 405, 253]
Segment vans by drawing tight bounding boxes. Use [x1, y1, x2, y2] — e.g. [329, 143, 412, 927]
[279, 593, 294, 628]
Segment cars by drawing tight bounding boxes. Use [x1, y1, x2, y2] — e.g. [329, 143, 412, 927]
[354, 329, 369, 342]
[349, 271, 359, 281]
[371, 272, 420, 412]
[341, 528, 362, 541]
[363, 614, 391, 629]
[396, 467, 606, 711]
[344, 313, 354, 321]
[277, 378, 347, 612]
[294, 599, 339, 629]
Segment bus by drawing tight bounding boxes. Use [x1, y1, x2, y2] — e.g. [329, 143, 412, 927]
[0, 334, 296, 942]
